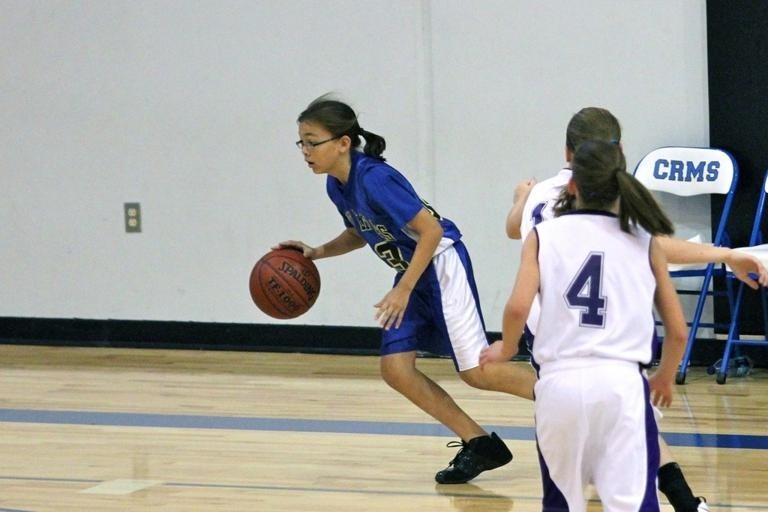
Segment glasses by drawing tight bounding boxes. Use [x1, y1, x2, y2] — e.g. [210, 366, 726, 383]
[296, 136, 342, 152]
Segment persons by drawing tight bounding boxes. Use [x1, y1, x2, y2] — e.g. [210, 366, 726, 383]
[478, 139, 687, 512]
[505, 106, 768, 510]
[271, 101, 538, 484]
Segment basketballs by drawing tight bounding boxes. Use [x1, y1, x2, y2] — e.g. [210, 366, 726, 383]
[250, 249, 320, 319]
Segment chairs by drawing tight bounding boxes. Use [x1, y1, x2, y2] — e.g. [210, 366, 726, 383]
[632, 145, 766, 386]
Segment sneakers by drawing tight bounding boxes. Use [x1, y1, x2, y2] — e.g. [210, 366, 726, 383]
[435, 432, 513, 485]
